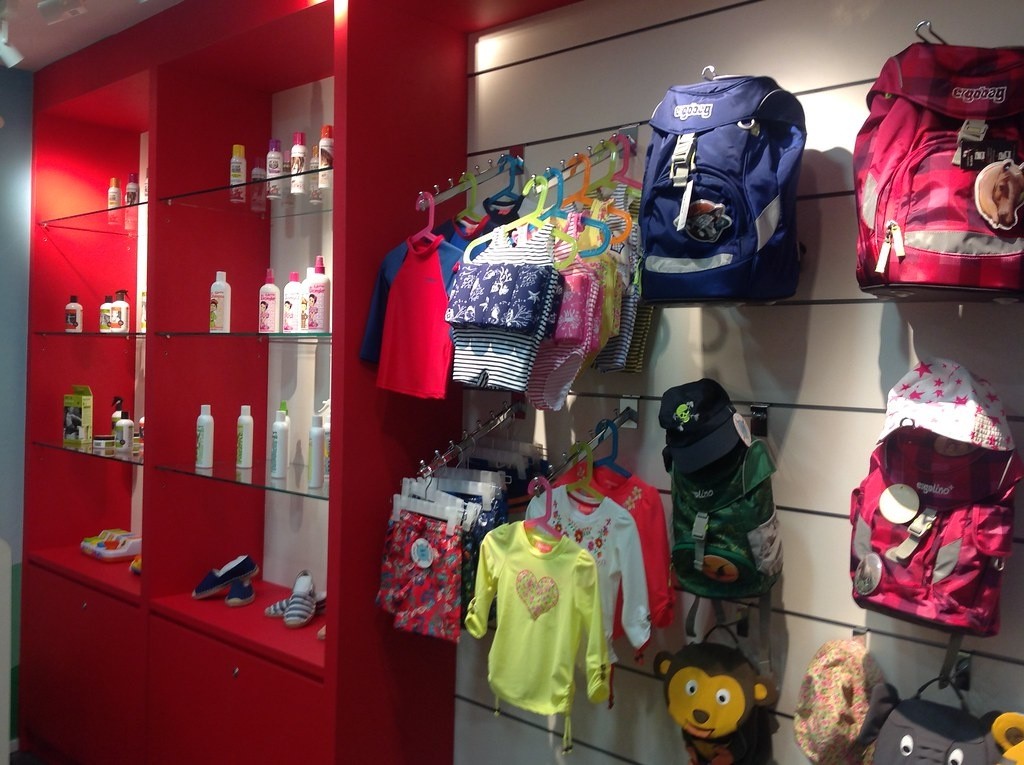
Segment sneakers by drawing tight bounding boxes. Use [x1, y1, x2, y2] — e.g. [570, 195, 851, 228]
[192, 553, 259, 600]
[264, 591, 327, 618]
[224, 579, 255, 606]
[282, 569, 318, 628]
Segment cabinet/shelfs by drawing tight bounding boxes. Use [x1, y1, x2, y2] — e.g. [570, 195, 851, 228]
[16, 0, 470, 765]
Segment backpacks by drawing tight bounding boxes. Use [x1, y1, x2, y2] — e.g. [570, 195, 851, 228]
[855, 678, 1014, 765]
[651, 624, 777, 764]
[852, 42, 1024, 295]
[848, 426, 1018, 691]
[637, 75, 807, 306]
[670, 433, 783, 648]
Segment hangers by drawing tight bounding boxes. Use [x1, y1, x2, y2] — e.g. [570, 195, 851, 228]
[411, 188, 439, 246]
[566, 441, 605, 502]
[455, 131, 643, 272]
[390, 408, 561, 542]
[592, 418, 633, 480]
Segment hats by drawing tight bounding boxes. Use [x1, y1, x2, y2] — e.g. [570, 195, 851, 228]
[878, 356, 1016, 452]
[658, 378, 744, 477]
[792, 635, 890, 765]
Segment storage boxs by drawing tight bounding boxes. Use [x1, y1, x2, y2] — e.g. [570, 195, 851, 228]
[62, 385, 95, 445]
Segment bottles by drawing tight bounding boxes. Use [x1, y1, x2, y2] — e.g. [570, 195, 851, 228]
[251, 153, 267, 211]
[108, 178, 122, 224]
[302, 267, 315, 329]
[65, 296, 82, 333]
[307, 415, 326, 488]
[266, 138, 283, 200]
[209, 270, 231, 334]
[236, 405, 253, 469]
[125, 172, 139, 230]
[100, 296, 113, 334]
[195, 405, 214, 468]
[270, 411, 288, 478]
[278, 400, 290, 467]
[310, 145, 322, 205]
[290, 131, 309, 196]
[230, 144, 247, 203]
[307, 255, 329, 334]
[114, 411, 134, 452]
[110, 290, 130, 333]
[283, 272, 302, 334]
[139, 416, 144, 458]
[258, 268, 280, 333]
[281, 150, 295, 208]
[318, 124, 334, 189]
